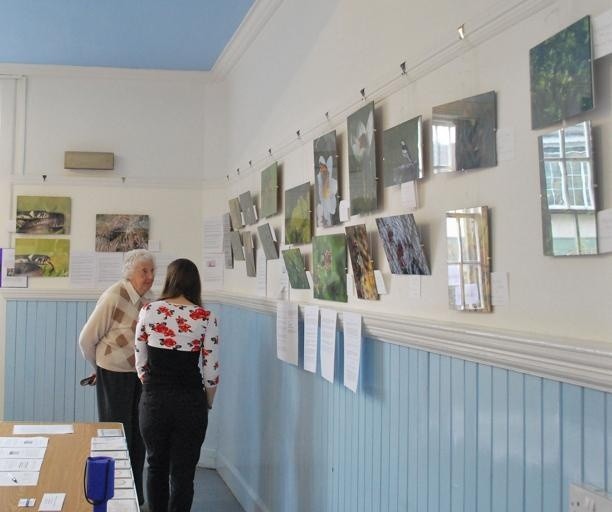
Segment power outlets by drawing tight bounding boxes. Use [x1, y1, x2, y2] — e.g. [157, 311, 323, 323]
[569, 484, 612, 512]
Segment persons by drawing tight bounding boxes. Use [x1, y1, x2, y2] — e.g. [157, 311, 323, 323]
[134, 258, 221, 512]
[78, 252, 156, 423]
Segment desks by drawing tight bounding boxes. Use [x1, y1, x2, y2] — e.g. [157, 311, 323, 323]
[0, 421, 141, 512]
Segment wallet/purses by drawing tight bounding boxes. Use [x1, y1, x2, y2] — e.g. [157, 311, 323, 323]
[80, 376, 94, 386]
